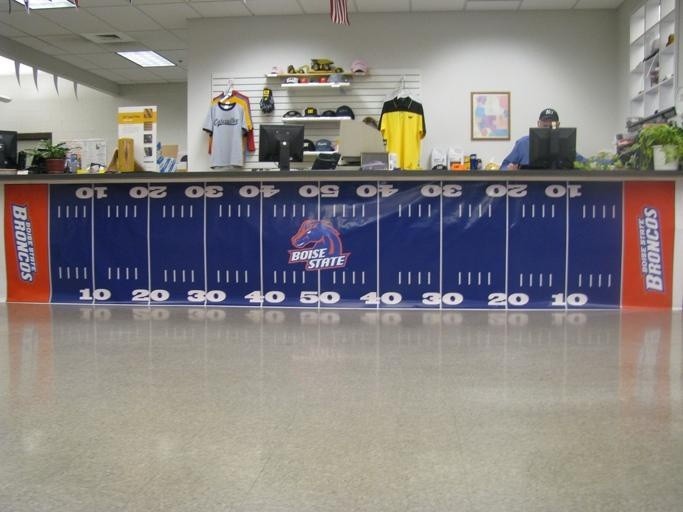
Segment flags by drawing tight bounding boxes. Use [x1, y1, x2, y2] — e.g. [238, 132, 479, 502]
[330, 0, 350, 27]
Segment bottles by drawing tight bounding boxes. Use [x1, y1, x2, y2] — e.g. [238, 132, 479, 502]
[470, 153, 477, 169]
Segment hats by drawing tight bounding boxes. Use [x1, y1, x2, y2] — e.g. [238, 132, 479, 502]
[336, 106, 354, 120]
[351, 58, 368, 72]
[303, 139, 315, 151]
[321, 110, 336, 117]
[315, 139, 332, 151]
[302, 105, 321, 116]
[540, 109, 558, 121]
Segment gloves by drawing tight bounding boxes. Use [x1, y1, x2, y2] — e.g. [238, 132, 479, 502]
[260, 90, 274, 113]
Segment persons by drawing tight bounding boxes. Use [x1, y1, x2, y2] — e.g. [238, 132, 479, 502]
[500, 106, 617, 170]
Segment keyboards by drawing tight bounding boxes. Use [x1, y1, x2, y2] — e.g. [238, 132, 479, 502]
[520, 165, 529, 169]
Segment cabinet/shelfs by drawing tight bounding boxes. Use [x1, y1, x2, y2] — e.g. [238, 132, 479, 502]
[281, 82, 353, 157]
[625, 0, 680, 133]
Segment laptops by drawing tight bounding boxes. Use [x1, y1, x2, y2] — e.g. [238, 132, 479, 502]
[311, 153, 341, 171]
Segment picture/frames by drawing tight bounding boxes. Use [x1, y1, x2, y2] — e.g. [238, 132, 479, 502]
[471, 92, 510, 140]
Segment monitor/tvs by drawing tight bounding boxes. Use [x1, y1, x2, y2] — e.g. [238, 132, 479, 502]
[340, 119, 386, 164]
[259, 125, 304, 173]
[0, 131, 17, 168]
[529, 128, 576, 170]
